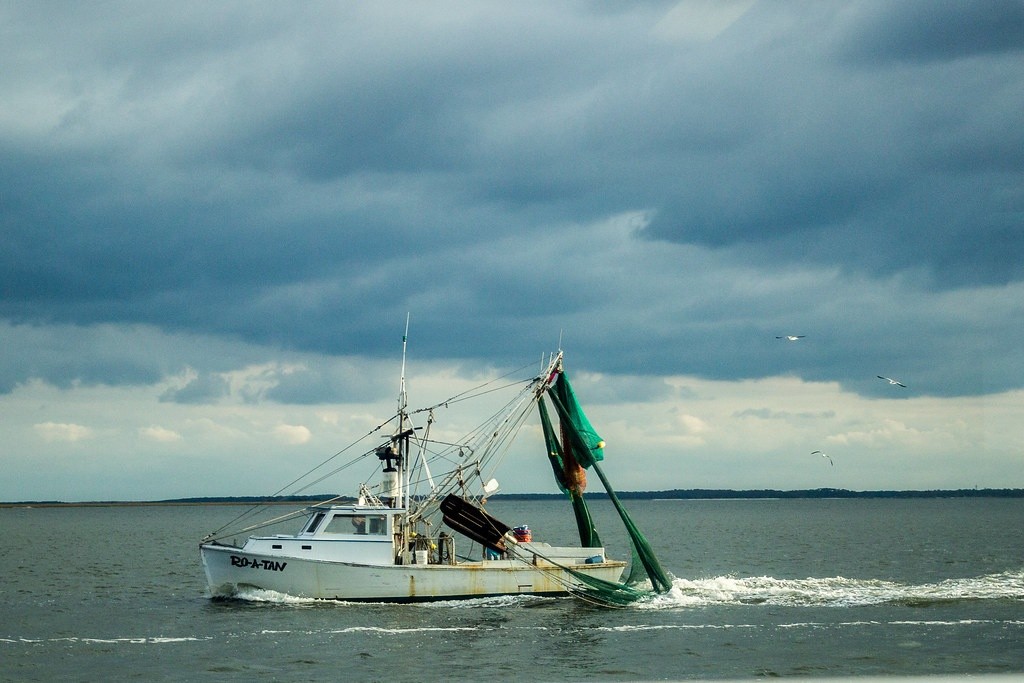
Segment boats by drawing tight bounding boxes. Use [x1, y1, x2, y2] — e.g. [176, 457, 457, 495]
[199, 310, 628, 597]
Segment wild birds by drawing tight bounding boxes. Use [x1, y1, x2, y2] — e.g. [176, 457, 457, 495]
[776, 336, 806, 341]
[811, 451, 834, 466]
[877, 375, 907, 388]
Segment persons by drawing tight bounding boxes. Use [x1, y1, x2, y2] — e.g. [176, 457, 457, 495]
[483, 546, 505, 561]
